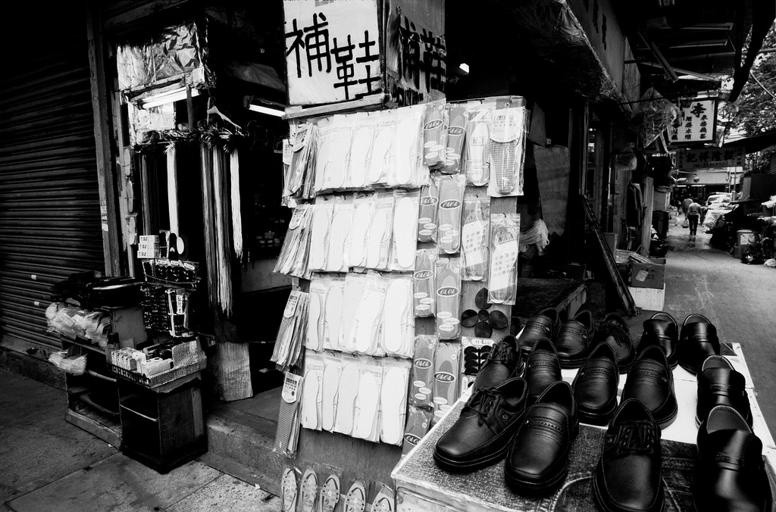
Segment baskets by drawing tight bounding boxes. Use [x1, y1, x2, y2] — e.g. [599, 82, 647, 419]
[110, 361, 201, 390]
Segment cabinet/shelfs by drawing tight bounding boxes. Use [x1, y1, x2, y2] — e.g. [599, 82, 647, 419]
[58, 338, 210, 477]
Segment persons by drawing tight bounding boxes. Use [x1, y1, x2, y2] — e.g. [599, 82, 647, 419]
[685, 198, 704, 237]
[682, 192, 695, 227]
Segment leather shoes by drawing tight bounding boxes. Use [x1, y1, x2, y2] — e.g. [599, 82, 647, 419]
[433, 377, 535, 474]
[522, 337, 562, 404]
[697, 405, 773, 512]
[517, 307, 720, 374]
[695, 354, 753, 429]
[472, 335, 524, 391]
[620, 345, 678, 430]
[571, 341, 619, 426]
[592, 398, 666, 512]
[504, 381, 580, 498]
